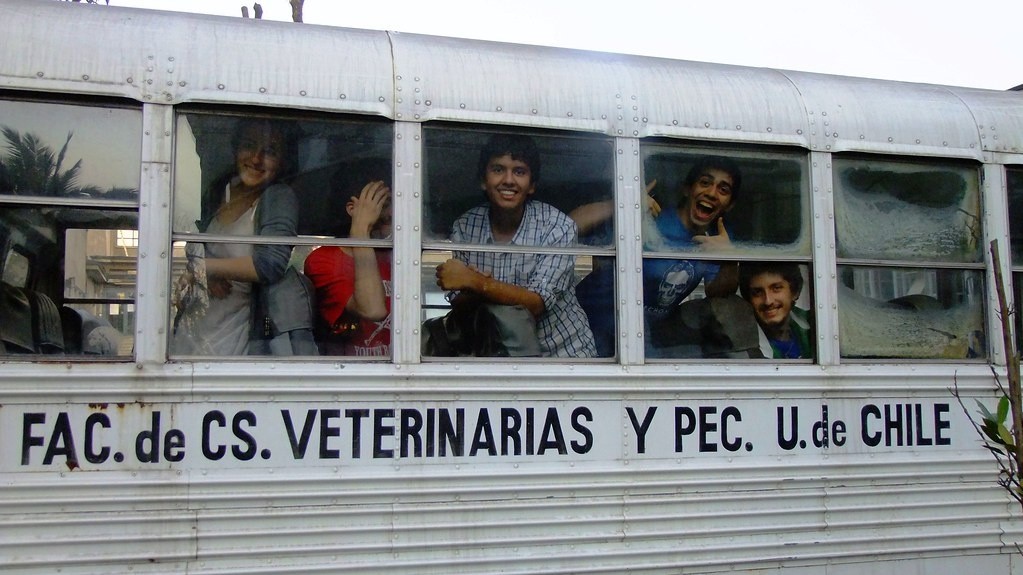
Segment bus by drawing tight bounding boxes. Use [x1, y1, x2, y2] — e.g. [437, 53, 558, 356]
[0, 1, 1023, 575]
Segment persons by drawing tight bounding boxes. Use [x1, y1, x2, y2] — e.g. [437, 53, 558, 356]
[169, 117, 299, 358]
[302, 156, 392, 354]
[738, 261, 814, 357]
[570, 153, 759, 357]
[434, 134, 598, 358]
